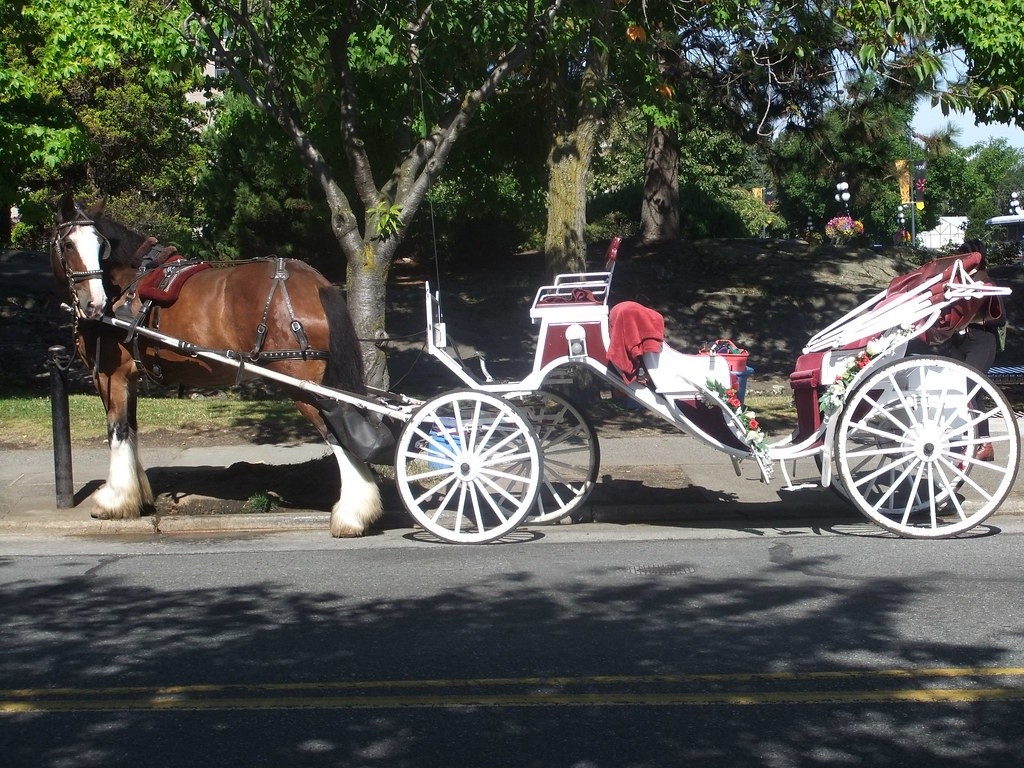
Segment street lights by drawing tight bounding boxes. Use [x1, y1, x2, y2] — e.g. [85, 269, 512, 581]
[835, 181, 853, 220]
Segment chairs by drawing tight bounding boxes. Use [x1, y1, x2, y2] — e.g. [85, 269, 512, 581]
[529, 237, 622, 324]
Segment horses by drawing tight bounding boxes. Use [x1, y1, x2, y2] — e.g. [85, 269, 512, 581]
[50, 190, 383, 538]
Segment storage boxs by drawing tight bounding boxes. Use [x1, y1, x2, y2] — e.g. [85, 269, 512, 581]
[698, 347, 756, 404]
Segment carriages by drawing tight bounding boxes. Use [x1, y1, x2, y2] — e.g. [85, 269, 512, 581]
[41, 187, 1023, 543]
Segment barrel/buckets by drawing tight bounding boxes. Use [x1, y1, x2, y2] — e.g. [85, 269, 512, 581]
[427, 430, 464, 472]
[697, 339, 755, 406]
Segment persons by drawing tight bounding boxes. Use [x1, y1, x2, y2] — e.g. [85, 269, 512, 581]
[943, 240, 1006, 460]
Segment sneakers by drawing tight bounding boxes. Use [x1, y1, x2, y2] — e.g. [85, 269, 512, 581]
[975, 436, 994, 462]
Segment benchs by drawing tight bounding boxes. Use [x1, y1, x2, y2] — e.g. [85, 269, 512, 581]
[605, 301, 733, 400]
[790, 293, 926, 437]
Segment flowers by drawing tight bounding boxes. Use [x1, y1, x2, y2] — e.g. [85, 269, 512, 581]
[704, 377, 776, 484]
[818, 324, 914, 412]
